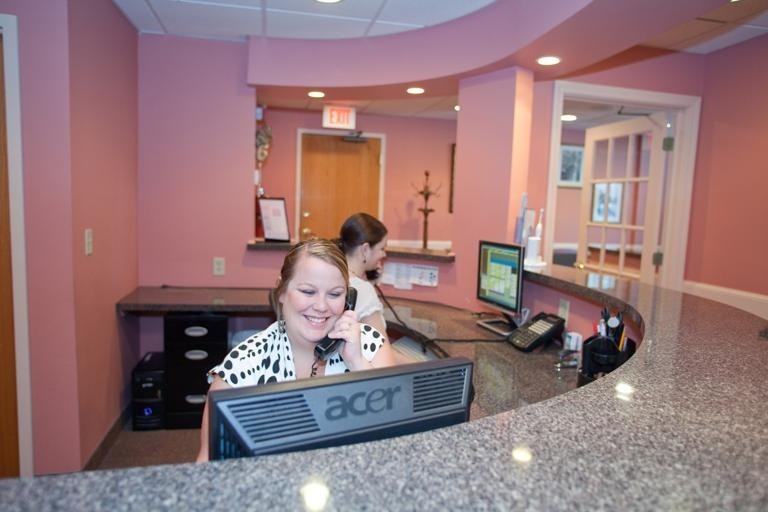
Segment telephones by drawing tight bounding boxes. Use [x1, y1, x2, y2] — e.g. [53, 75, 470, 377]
[314, 287, 357, 359]
[366, 270, 378, 280]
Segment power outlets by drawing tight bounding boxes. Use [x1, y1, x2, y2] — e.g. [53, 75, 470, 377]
[213, 258, 226, 278]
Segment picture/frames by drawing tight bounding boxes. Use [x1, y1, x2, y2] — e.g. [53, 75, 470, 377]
[591, 180, 624, 224]
[257, 197, 291, 242]
[558, 143, 585, 190]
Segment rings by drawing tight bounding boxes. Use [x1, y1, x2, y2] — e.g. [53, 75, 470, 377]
[348, 323, 351, 327]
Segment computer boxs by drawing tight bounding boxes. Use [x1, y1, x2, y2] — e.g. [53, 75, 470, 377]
[131, 351, 168, 430]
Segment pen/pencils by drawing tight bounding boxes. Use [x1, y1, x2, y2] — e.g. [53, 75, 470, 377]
[592, 306, 628, 352]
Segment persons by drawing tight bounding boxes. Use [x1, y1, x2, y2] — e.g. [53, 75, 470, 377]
[196, 237, 396, 465]
[330, 212, 408, 363]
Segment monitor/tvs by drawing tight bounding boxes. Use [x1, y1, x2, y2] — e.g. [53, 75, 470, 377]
[476, 239, 526, 336]
[207, 355, 474, 460]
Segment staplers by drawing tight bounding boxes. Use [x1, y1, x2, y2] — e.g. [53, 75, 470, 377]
[554, 350, 578, 368]
